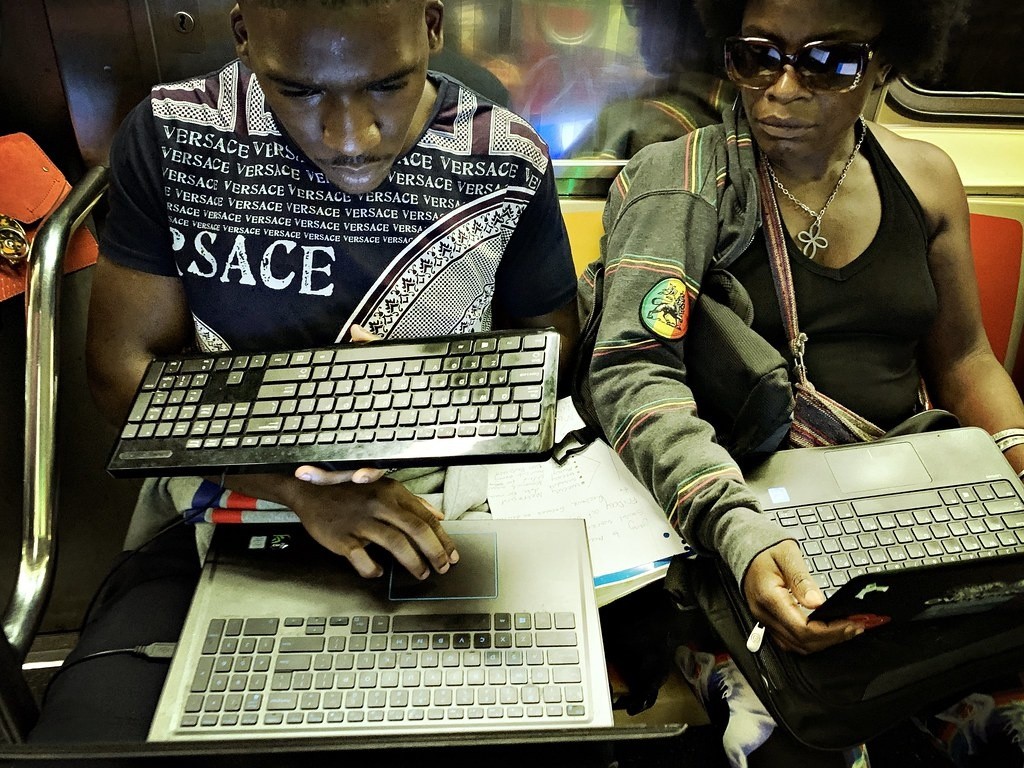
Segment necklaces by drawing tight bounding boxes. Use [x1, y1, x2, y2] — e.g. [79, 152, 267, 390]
[756, 113, 868, 260]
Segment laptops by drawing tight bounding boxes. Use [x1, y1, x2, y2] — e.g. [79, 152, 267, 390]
[0, 518, 688, 768]
[742, 426, 1024, 626]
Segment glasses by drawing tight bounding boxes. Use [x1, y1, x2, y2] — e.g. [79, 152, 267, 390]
[723, 29, 885, 95]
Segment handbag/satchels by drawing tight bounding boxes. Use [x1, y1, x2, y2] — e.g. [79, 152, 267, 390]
[685, 549, 1024, 750]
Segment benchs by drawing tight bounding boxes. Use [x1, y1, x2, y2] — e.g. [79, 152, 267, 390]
[559, 197, 1024, 731]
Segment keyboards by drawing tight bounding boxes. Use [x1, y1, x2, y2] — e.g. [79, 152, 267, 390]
[104, 325, 563, 479]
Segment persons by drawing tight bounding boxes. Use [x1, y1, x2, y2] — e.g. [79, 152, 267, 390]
[84, 0, 580, 582]
[557, 0, 741, 159]
[578, 0, 1024, 767]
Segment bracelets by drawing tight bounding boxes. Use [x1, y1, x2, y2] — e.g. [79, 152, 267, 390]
[1018, 470, 1024, 479]
[991, 428, 1024, 452]
